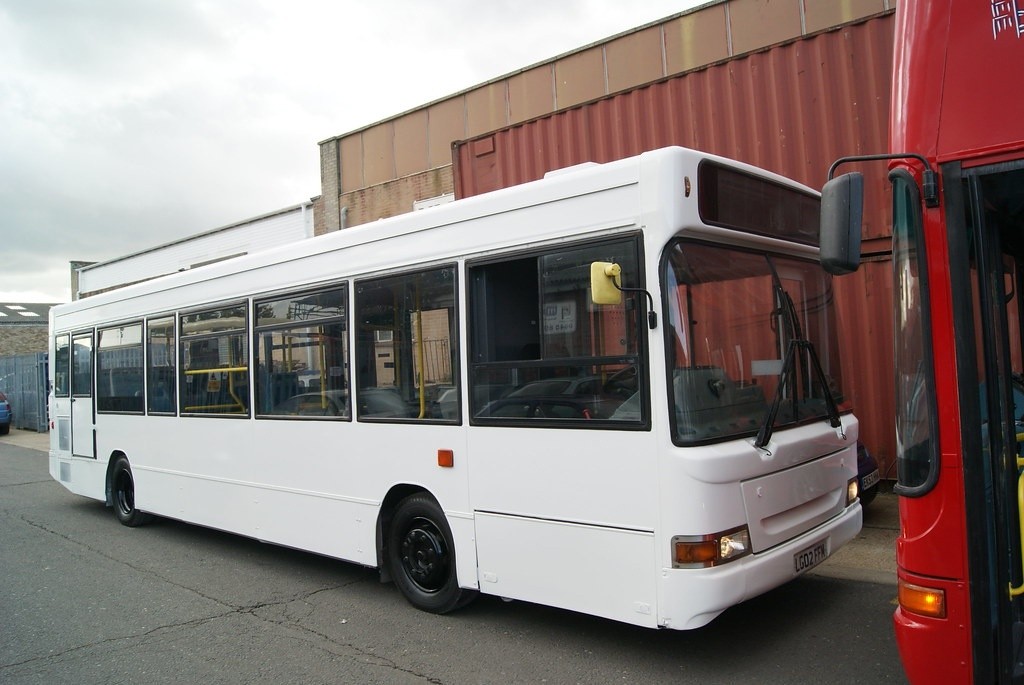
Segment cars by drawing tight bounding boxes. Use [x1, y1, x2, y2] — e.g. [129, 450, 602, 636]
[0, 391, 11, 435]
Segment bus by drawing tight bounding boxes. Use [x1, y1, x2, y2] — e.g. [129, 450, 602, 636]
[48, 145, 862, 631]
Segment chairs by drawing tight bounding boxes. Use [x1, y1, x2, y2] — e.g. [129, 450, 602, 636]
[110, 368, 137, 397]
[265, 372, 299, 416]
[152, 382, 176, 411]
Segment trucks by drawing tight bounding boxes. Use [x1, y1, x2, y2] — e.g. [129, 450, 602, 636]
[820, 0, 1024, 685]
